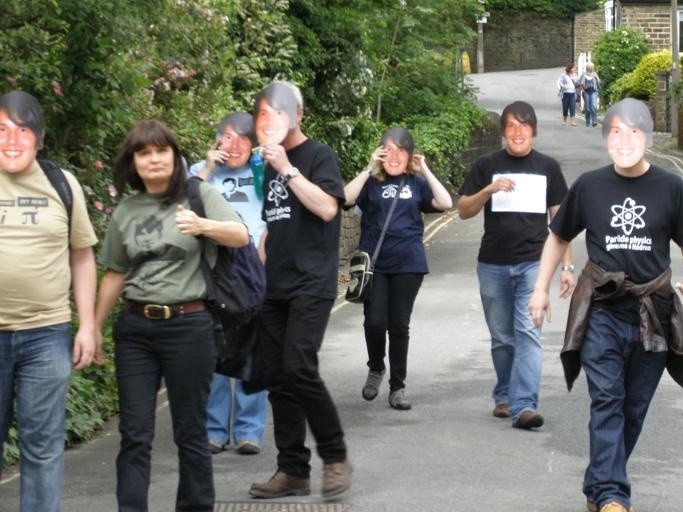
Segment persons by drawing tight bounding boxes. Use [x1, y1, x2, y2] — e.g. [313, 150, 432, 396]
[380, 127, 414, 176]
[216, 112, 257, 169]
[501, 101, 537, 154]
[459, 100, 577, 430]
[2, 91, 45, 172]
[254, 84, 297, 146]
[602, 98, 655, 168]
[578, 61, 603, 126]
[558, 62, 580, 122]
[190, 111, 269, 453]
[2, 90, 99, 509]
[92, 120, 248, 508]
[526, 96, 682, 512]
[249, 80, 355, 502]
[341, 126, 451, 410]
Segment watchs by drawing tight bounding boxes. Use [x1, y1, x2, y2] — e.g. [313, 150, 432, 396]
[561, 264, 572, 272]
[285, 167, 299, 179]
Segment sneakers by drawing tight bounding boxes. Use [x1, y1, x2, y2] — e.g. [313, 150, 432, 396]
[601, 501, 634, 512]
[362, 366, 386, 400]
[250, 471, 310, 498]
[586, 500, 600, 512]
[235, 441, 259, 455]
[390, 388, 413, 409]
[210, 441, 223, 453]
[494, 404, 510, 417]
[323, 461, 349, 498]
[512, 411, 543, 428]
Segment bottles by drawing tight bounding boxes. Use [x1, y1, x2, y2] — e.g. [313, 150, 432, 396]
[247, 147, 268, 199]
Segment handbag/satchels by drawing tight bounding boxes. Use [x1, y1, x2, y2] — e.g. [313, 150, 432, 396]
[205, 222, 267, 324]
[346, 252, 373, 302]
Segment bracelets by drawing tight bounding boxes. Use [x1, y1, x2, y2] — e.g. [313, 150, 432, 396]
[363, 168, 372, 175]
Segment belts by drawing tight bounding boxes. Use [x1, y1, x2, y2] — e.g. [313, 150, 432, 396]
[125, 298, 206, 319]
